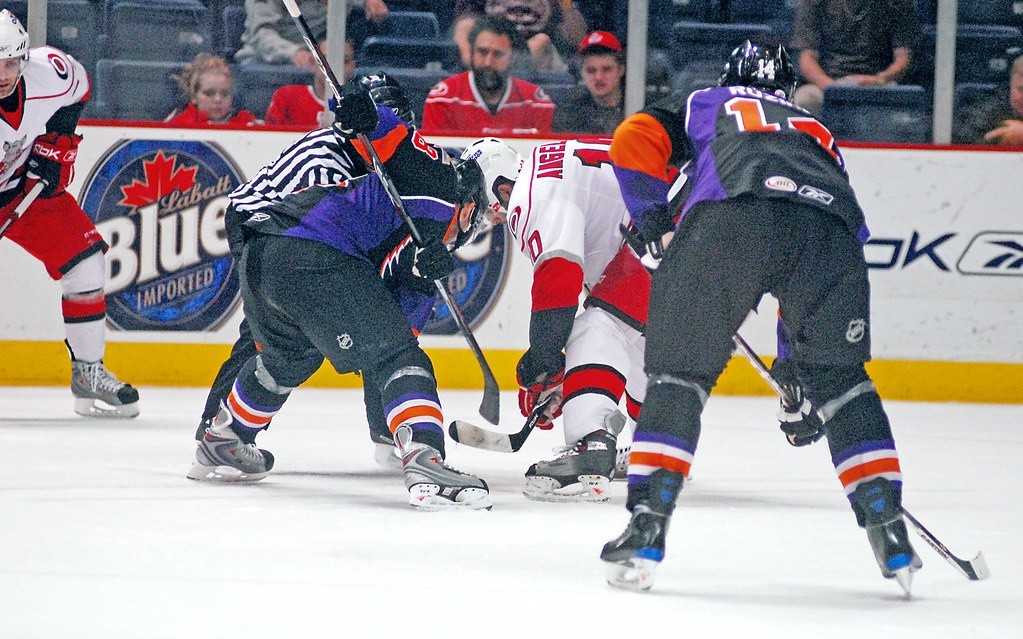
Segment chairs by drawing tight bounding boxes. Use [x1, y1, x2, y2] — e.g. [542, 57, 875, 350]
[47, 0, 1023, 150]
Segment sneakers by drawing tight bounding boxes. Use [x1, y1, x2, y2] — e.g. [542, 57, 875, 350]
[187, 395, 276, 484]
[71, 360, 141, 419]
[369, 428, 404, 470]
[402, 441, 490, 511]
[849, 478, 921, 597]
[523, 430, 618, 503]
[602, 507, 670, 593]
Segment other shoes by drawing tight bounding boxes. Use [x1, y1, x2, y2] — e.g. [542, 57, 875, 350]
[612, 447, 633, 480]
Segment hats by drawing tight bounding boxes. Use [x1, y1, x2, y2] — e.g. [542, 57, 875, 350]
[576, 31, 624, 54]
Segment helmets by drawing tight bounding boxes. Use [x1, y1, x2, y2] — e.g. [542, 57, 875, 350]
[0, 8, 31, 61]
[453, 156, 487, 246]
[717, 39, 799, 103]
[459, 137, 525, 213]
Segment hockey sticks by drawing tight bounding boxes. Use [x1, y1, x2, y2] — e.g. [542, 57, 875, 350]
[448, 388, 561, 451]
[620, 223, 991, 584]
[284, 0, 502, 426]
[0, 174, 49, 239]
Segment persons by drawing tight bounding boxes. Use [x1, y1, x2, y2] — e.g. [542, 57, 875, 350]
[551, 31, 626, 132]
[599, 35, 924, 579]
[792, 0, 911, 120]
[233, 0, 389, 71]
[462, 138, 676, 498]
[163, 52, 257, 125]
[454, 0, 570, 72]
[421, 12, 557, 129]
[195, 94, 489, 505]
[0, 8, 141, 405]
[264, 30, 355, 125]
[953, 54, 1023, 146]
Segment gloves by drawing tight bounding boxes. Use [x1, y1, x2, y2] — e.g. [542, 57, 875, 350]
[640, 238, 667, 276]
[402, 239, 453, 284]
[331, 82, 380, 137]
[516, 349, 565, 391]
[24, 132, 82, 199]
[516, 384, 564, 429]
[516, 389, 563, 429]
[779, 399, 825, 446]
